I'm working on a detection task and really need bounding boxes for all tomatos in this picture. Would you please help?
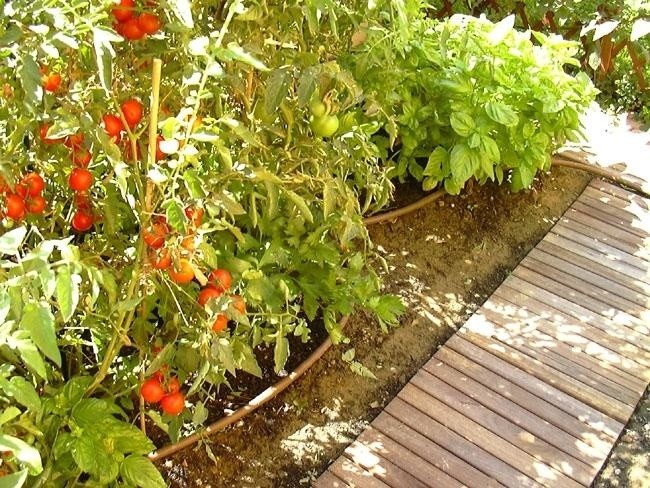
[0,0,248,415]
[307,97,339,140]
